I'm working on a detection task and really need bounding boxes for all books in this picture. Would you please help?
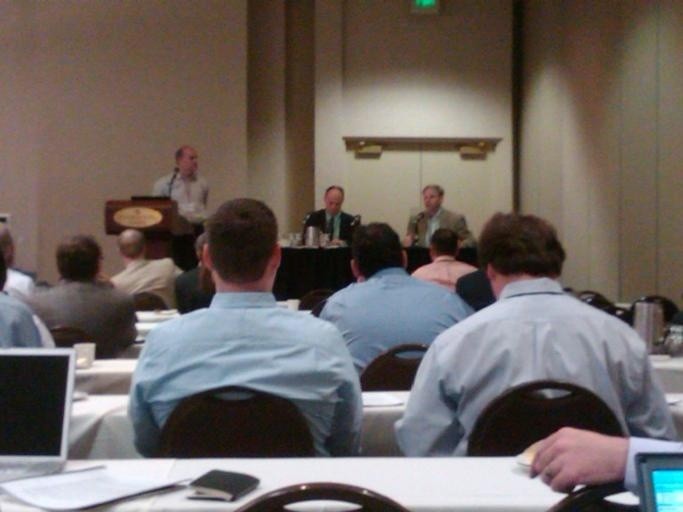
[188,469,259,502]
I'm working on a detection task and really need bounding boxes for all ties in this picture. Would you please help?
[633,451,683,511]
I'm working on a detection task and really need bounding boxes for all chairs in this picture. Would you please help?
[0,239,683,512]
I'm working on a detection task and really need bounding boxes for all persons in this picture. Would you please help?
[404,184,473,247]
[149,147,210,234]
[302,186,354,247]
[173,235,217,314]
[412,228,476,293]
[0,222,42,349]
[529,427,683,496]
[28,232,138,361]
[319,222,476,377]
[111,230,180,310]
[394,209,676,457]
[128,198,363,459]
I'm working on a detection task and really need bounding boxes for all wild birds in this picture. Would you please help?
[0,348,77,483]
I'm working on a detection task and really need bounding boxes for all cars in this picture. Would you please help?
[305,225,319,248]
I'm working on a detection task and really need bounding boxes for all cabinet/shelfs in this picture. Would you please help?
[633,295,665,354]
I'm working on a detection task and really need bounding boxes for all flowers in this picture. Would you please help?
[300,208,311,250]
[350,214,363,246]
[168,166,180,200]
[412,211,425,246]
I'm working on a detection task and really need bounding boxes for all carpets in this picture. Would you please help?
[326,216,334,241]
